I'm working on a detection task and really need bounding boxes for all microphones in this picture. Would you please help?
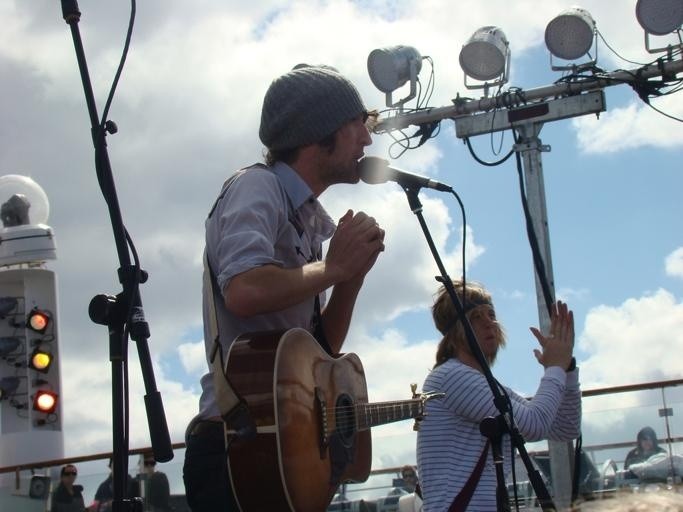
[357,154,453,194]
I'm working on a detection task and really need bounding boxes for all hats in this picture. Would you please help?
[259,63,368,151]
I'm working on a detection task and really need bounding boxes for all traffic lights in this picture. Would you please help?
[27,309,58,431]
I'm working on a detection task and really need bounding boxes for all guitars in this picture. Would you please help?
[222,327,445,511]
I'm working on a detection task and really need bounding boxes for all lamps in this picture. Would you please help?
[366,1,683,109]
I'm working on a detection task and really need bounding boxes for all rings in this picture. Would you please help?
[548,334,554,338]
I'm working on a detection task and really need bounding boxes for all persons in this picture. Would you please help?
[181,64,387,511]
[93,457,136,498]
[50,464,84,512]
[400,463,417,483]
[625,425,668,479]
[133,452,168,502]
[414,278,581,512]
